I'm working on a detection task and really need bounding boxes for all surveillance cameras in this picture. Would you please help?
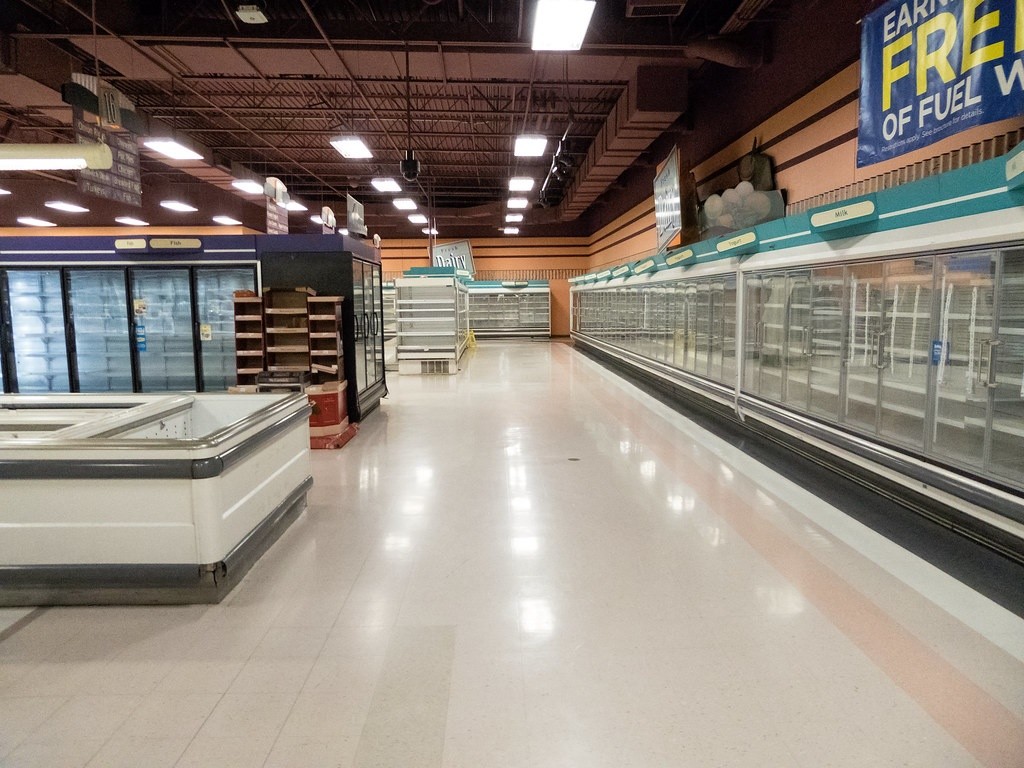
[400,160,420,181]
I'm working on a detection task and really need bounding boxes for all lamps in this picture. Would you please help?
[1,1,596,238]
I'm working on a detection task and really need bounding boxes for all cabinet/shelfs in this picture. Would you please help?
[230,287,349,435]
